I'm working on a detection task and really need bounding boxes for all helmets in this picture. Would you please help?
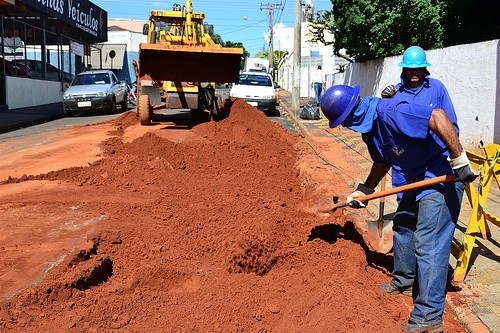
[321,82,360,128]
[398,46,431,68]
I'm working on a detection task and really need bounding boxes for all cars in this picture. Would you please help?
[11,59,74,83]
[229,71,281,115]
[0,57,31,78]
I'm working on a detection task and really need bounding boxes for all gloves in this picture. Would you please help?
[447,150,478,184]
[381,84,397,98]
[346,183,375,209]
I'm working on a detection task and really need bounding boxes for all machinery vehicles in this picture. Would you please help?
[132,0,243,124]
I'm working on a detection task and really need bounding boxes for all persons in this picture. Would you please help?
[382,46,460,206]
[311,65,326,106]
[321,85,478,333]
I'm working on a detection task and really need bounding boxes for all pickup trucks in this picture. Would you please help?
[62,69,128,116]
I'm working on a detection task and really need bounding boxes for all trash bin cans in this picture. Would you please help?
[314,82,322,104]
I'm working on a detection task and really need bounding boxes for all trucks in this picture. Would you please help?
[245,57,270,85]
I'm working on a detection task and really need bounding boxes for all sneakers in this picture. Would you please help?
[377,283,413,296]
[401,320,443,333]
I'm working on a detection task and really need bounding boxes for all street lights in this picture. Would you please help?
[244,16,270,28]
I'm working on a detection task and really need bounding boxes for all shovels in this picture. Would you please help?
[318,173,457,213]
[367,173,394,255]
